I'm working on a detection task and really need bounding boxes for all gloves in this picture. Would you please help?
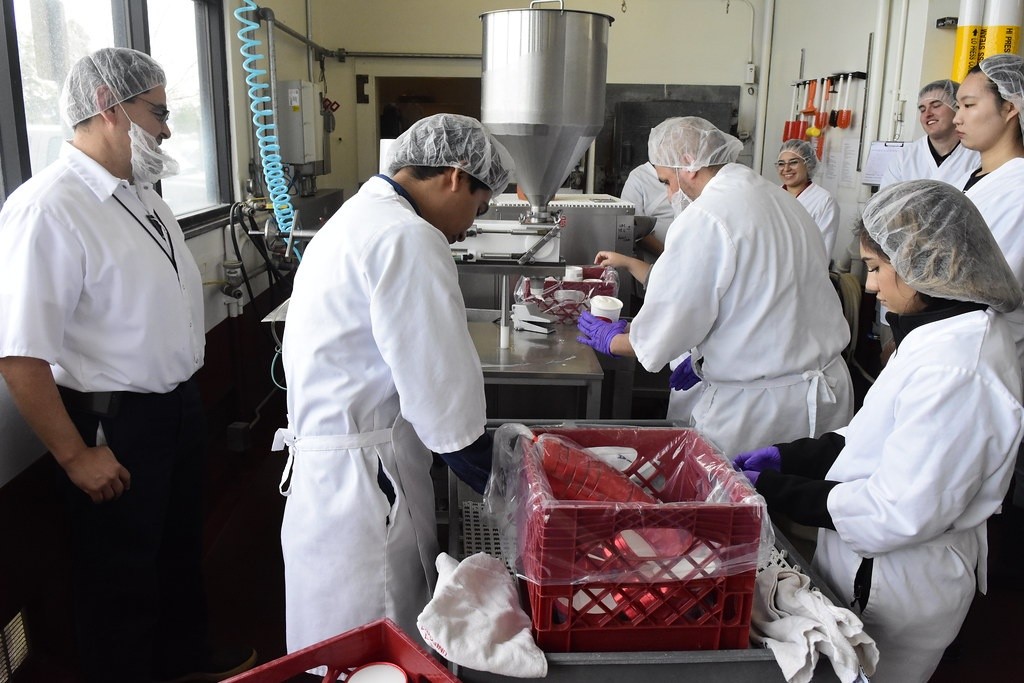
[731,446,782,487]
[576,312,627,360]
[669,355,701,390]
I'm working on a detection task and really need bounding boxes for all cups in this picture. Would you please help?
[344,662,408,683]
[589,295,624,323]
[564,265,583,282]
[583,278,603,282]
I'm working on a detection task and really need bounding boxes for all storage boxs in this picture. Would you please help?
[218,618,464,683]
[525,266,615,323]
[522,428,763,654]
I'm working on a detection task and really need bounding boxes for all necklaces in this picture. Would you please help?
[109,187,179,275]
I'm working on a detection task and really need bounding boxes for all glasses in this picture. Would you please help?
[133,96,169,124]
[774,158,802,170]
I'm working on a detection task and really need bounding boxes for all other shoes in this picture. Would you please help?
[171,641,257,683]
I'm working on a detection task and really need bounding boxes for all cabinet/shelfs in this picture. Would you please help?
[449,321,605,556]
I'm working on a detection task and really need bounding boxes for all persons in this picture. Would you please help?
[279,112,510,683]
[876,77,982,372]
[771,138,840,271]
[951,54,1024,300]
[620,150,696,270]
[730,179,1024,683]
[1,47,260,683]
[578,116,853,483]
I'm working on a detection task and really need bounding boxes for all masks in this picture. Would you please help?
[669,166,693,220]
[118,103,179,186]
